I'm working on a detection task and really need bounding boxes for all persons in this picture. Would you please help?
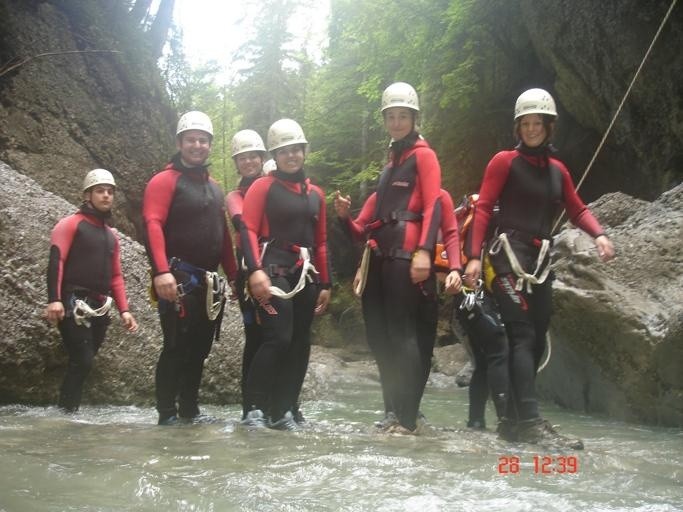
[464,88,617,448]
[334,132,464,425]
[45,169,139,413]
[140,109,239,423]
[238,119,331,429]
[226,130,306,420]
[354,81,440,434]
[452,194,512,431]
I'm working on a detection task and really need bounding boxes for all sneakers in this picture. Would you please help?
[240,403,310,434]
[373,410,437,437]
[463,414,584,452]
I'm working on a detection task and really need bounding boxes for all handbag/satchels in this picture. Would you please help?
[434,194,479,270]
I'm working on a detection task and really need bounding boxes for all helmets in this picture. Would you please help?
[381,83,420,111]
[267,119,309,153]
[514,88,557,121]
[176,111,213,137]
[82,169,116,192]
[231,130,266,157]
